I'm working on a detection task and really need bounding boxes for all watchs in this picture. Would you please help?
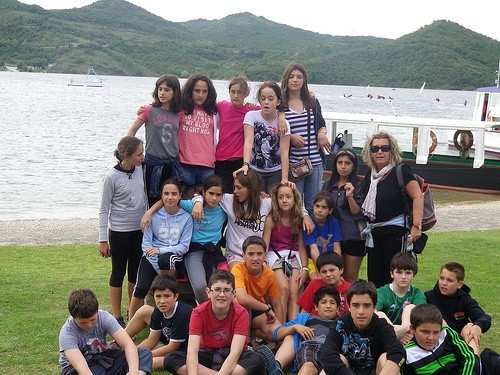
[413,224,423,230]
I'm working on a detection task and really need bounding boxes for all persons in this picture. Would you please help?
[211,78,289,189]
[163,270,265,375]
[319,278,407,375]
[58,289,154,375]
[232,80,296,194]
[402,303,484,375]
[358,130,424,287]
[374,251,427,327]
[127,74,182,206]
[230,234,282,342]
[132,273,195,372]
[271,286,343,375]
[139,168,343,308]
[423,261,493,357]
[275,62,332,223]
[296,251,353,322]
[137,73,221,199]
[322,147,369,284]
[96,135,150,331]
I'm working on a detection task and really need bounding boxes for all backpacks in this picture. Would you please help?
[397,163,436,231]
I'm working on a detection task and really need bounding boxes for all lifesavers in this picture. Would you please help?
[454,130,473,152]
[413,130,438,154]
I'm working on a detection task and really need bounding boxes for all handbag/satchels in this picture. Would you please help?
[288,157,314,180]
[282,259,293,278]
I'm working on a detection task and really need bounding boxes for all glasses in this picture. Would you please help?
[210,287,233,294]
[370,145,392,153]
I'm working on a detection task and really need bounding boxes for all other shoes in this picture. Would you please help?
[116,317,126,327]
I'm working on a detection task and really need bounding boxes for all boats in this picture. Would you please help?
[67,66,102,87]
[323,62,500,196]
